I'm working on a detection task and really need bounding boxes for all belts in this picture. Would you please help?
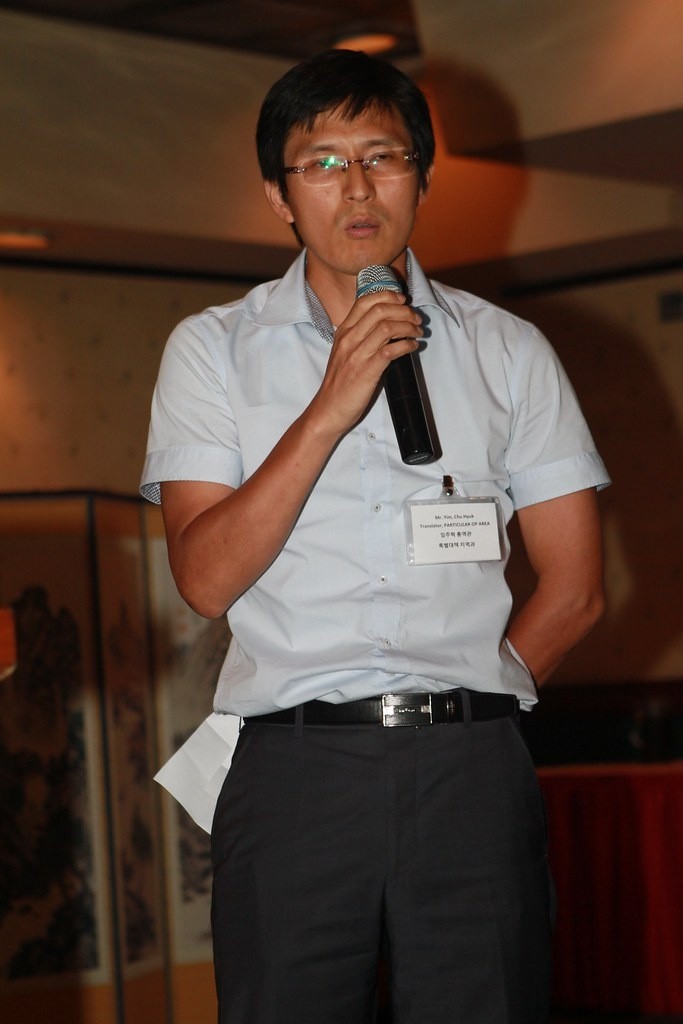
[242,687,521,731]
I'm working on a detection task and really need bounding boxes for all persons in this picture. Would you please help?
[140,50,612,1024]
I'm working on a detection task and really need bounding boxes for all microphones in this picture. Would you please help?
[356,265,434,464]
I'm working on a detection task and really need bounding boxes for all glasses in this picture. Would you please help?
[275,150,422,187]
